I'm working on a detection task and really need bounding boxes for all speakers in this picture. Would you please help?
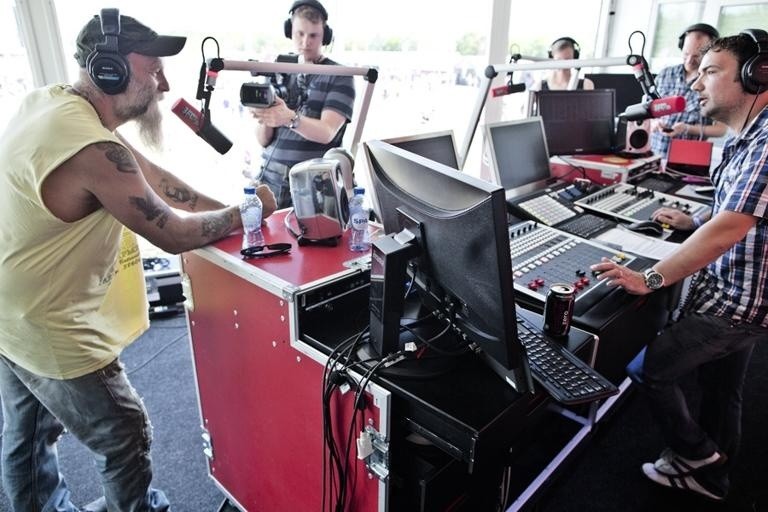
[614,115,652,155]
[286,156,352,245]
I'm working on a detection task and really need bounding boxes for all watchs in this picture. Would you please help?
[643,268,666,291]
[286,115,300,129]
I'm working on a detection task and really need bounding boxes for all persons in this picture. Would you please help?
[0,14,279,512]
[529,38,595,91]
[641,23,729,159]
[249,0,356,209]
[590,34,768,501]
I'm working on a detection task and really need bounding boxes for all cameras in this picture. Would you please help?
[239,53,312,110]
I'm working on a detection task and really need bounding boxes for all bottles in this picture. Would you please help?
[348,187,370,252]
[239,187,265,254]
[542,283,576,338]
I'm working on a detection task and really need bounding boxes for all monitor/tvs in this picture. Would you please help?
[355,140,536,396]
[379,129,461,172]
[537,88,617,155]
[483,116,554,201]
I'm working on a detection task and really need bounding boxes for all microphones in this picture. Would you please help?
[545,175,558,184]
[171,96,233,155]
[491,82,527,99]
[621,96,686,126]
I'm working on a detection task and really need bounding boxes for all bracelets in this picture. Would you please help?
[693,215,701,228]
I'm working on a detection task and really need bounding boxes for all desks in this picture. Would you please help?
[180,154,662,511]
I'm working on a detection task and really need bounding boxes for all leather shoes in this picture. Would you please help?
[652,448,728,478]
[641,461,729,503]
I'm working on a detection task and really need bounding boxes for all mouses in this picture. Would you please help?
[627,220,664,238]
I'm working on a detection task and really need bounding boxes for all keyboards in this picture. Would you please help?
[516,311,621,405]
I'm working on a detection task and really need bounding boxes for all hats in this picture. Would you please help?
[72,14,189,68]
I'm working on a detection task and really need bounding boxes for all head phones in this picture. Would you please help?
[677,23,719,49]
[85,8,131,95]
[739,28,768,95]
[282,0,333,47]
[547,37,580,59]
